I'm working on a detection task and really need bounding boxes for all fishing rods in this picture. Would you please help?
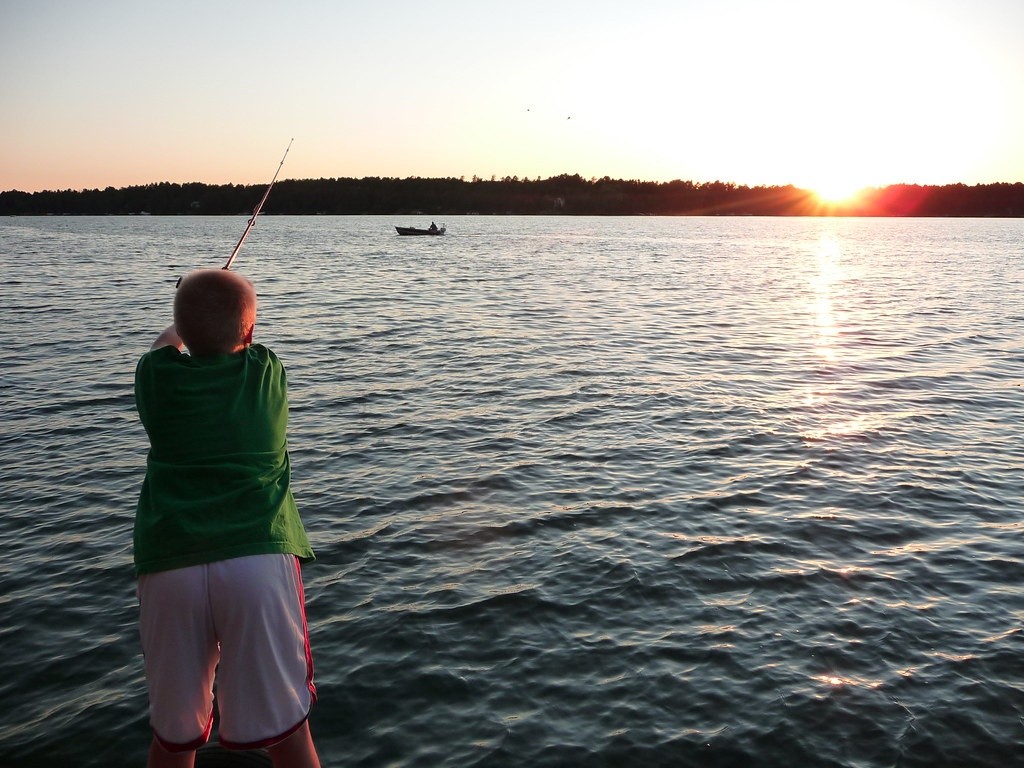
[222,137,294,274]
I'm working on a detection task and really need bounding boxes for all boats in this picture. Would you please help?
[394,226,446,236]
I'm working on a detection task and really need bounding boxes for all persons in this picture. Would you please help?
[132,268,321,768]
[429,222,438,231]
